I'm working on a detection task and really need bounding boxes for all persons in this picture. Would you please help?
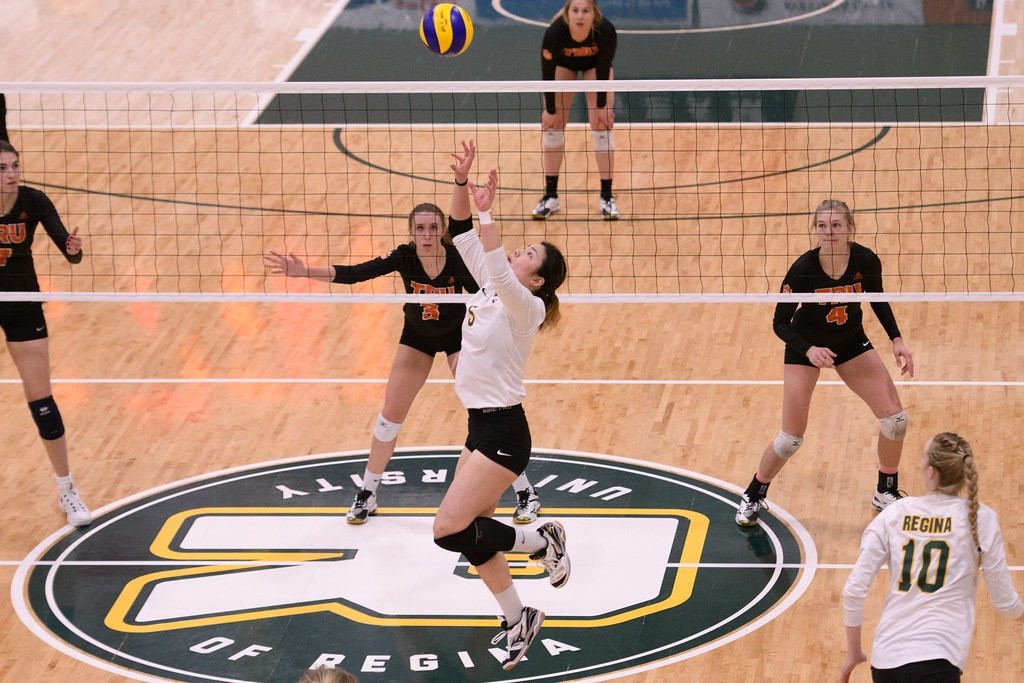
[531,0,621,220]
[735,199,913,526]
[0,93,92,527]
[837,432,1024,683]
[263,203,541,524]
[433,139,572,671]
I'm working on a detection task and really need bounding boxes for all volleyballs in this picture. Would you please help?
[419,3,475,57]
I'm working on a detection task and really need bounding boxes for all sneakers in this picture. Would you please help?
[513,486,541,524]
[346,486,377,524]
[532,196,560,219]
[871,483,909,511]
[600,196,620,219]
[735,492,769,527]
[529,520,571,589]
[491,606,545,671]
[59,490,92,526]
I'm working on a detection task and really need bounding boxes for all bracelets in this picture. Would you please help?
[475,208,496,225]
[455,178,469,187]
